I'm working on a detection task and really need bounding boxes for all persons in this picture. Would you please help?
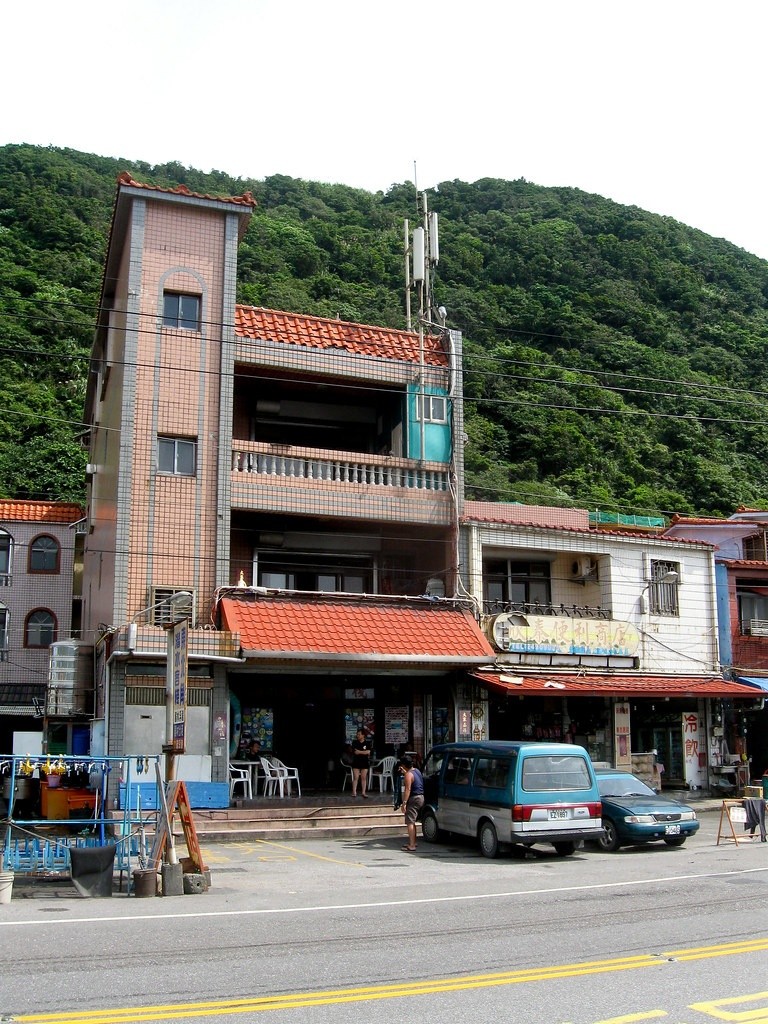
[399,755,425,852]
[351,729,371,798]
[244,739,274,795]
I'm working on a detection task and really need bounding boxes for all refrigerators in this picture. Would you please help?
[653,727,689,786]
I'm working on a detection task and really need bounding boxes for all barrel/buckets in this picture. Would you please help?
[45,637,97,717]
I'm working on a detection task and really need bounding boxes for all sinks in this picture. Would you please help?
[711,764,739,775]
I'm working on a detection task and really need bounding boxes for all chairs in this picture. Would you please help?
[340,759,369,793]
[271,757,302,799]
[260,757,289,799]
[367,757,397,794]
[229,764,252,800]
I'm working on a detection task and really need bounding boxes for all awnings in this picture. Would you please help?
[737,676,768,691]
[467,673,768,700]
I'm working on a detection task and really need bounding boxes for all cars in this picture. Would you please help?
[545,768,701,852]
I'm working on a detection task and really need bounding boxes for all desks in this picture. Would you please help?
[230,760,262,798]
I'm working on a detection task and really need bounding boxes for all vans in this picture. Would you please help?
[399,738,605,858]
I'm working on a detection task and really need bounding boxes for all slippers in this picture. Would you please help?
[401,844,418,851]
[351,792,369,798]
